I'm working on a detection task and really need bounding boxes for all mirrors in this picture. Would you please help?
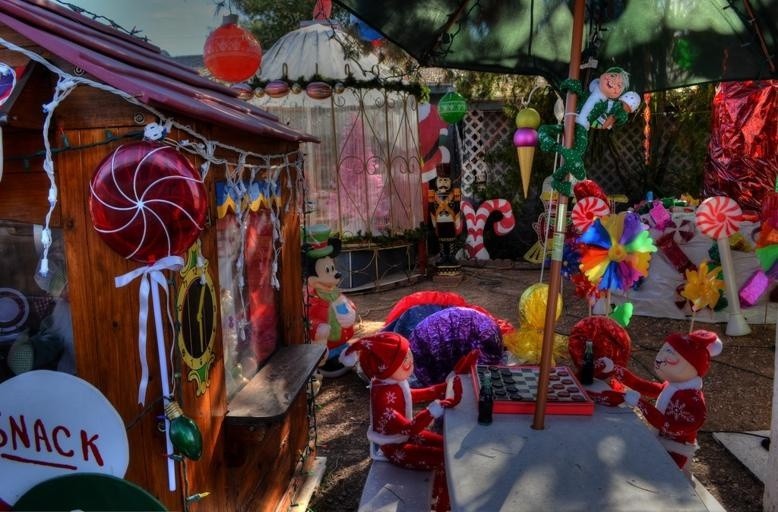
[177,239,219,398]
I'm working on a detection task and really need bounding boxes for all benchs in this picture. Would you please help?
[684,469,727,512]
[356,460,438,512]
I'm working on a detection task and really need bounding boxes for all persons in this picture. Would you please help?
[537,65,629,195]
[429,164,464,275]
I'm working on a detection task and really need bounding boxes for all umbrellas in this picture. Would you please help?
[333,0,778,430]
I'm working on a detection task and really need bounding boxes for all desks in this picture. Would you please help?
[444,362,710,512]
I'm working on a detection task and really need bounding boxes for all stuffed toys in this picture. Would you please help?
[595,330,726,471]
[342,330,464,511]
[302,223,360,378]
